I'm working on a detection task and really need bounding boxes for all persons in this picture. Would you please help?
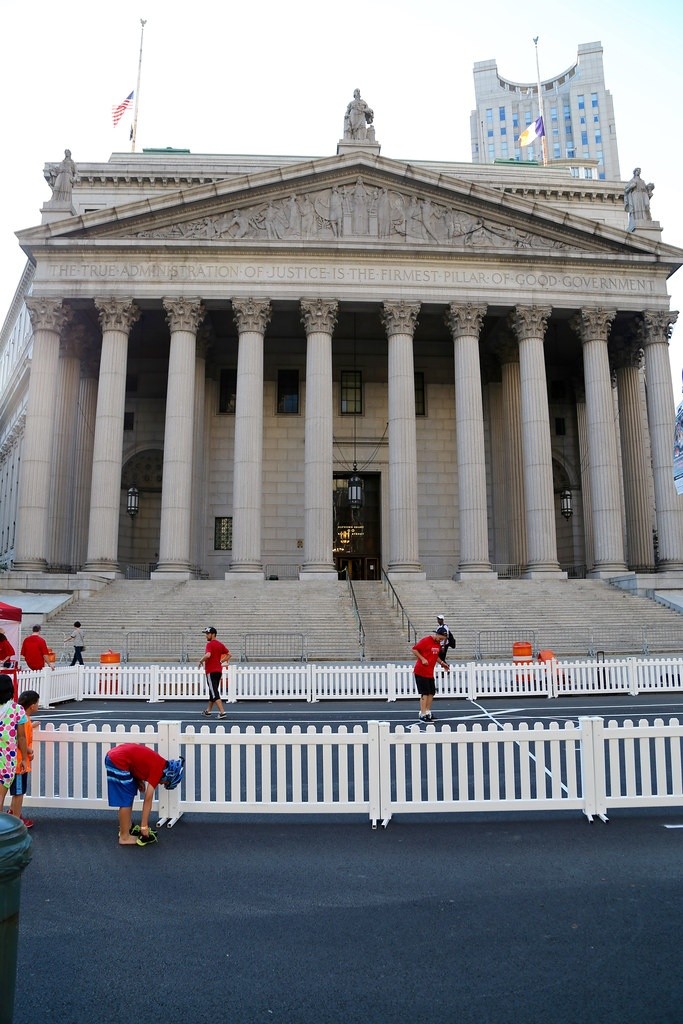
[197,627,232,719]
[344,88,374,140]
[105,742,185,845]
[0,633,15,669]
[412,628,451,722]
[64,621,86,665]
[436,615,449,668]
[7,690,40,827]
[624,167,655,224]
[0,674,27,812]
[50,148,77,204]
[20,624,55,671]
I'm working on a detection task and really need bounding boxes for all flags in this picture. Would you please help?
[112,90,135,128]
[518,116,545,147]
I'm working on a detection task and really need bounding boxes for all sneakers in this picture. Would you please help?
[136,831,159,846]
[129,825,157,836]
[214,712,227,719]
[201,710,211,717]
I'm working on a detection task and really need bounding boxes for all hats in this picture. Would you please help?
[437,615,445,619]
[202,627,217,634]
[0,628,5,635]
[436,627,447,637]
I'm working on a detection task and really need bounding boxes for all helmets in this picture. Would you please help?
[160,756,185,790]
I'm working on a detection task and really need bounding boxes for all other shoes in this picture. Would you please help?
[5,809,34,828]
[427,714,439,722]
[419,715,434,724]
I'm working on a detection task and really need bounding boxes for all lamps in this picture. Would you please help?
[560,376,574,522]
[348,311,363,510]
[127,395,140,520]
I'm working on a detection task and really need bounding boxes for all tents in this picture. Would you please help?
[0,602,22,670]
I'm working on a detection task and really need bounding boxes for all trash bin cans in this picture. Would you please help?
[511,641,534,685]
[99,649,122,695]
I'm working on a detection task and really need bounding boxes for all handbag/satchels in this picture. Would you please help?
[80,646,85,651]
[442,624,456,649]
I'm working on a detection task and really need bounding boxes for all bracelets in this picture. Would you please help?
[441,661,443,664]
[141,826,147,830]
